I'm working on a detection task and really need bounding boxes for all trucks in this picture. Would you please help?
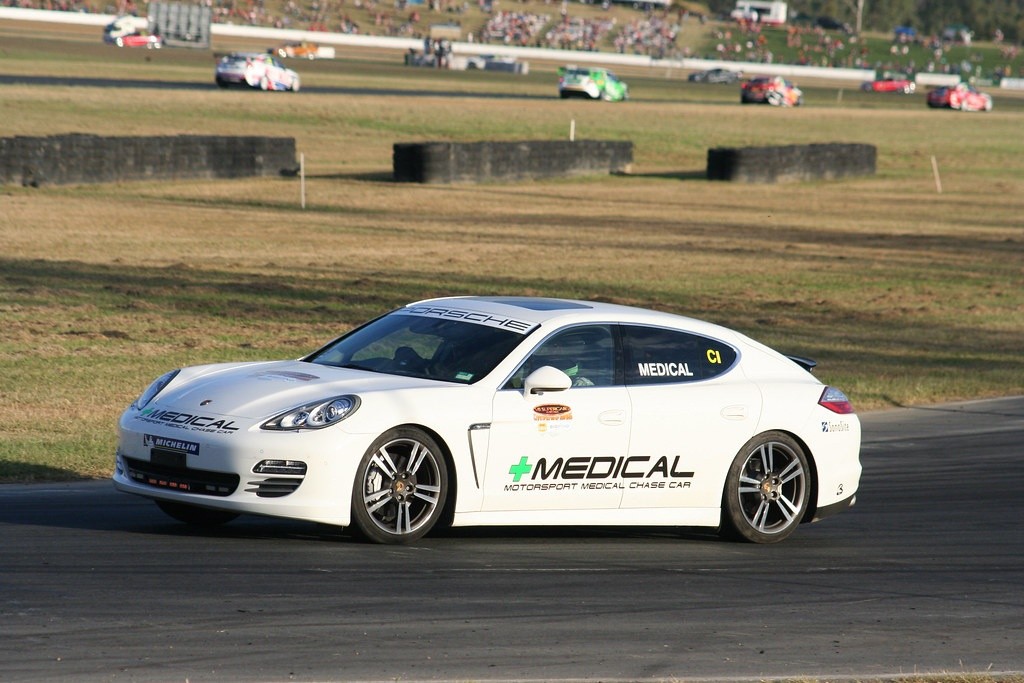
[729,0,787,26]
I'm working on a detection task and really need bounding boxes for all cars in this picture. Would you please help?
[405,49,485,71]
[113,295,865,542]
[273,44,318,60]
[214,50,302,93]
[687,68,737,86]
[861,75,914,95]
[105,29,162,49]
[742,75,804,105]
[926,82,993,112]
[558,66,630,102]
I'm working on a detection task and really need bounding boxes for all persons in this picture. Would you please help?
[504,334,595,387]
[0,1,1024,78]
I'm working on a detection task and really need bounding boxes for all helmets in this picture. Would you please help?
[529,333,579,380]
